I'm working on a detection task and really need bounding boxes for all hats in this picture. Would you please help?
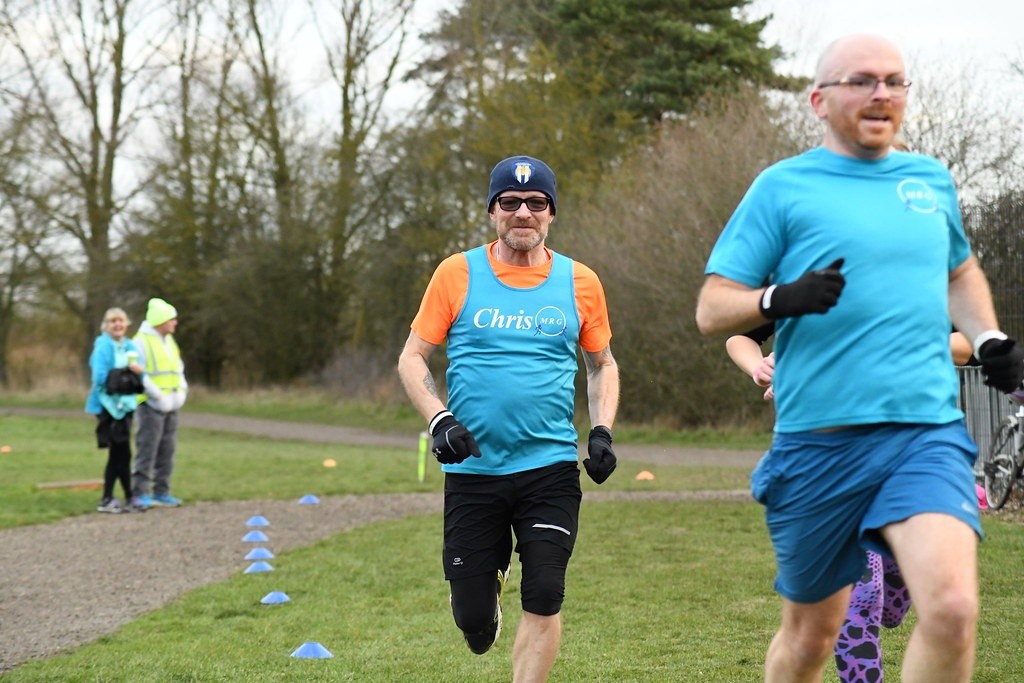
[487,155,556,215]
[146,297,177,327]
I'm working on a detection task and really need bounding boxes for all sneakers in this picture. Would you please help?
[97,497,130,513]
[125,496,148,513]
[141,495,163,507]
[153,494,184,507]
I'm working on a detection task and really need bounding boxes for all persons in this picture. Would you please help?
[88,305,150,515]
[127,298,191,513]
[722,276,972,683]
[396,150,621,682]
[693,32,1021,682]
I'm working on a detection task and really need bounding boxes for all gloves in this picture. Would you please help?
[973,330,1024,394]
[759,257,846,317]
[582,425,616,485]
[427,410,482,464]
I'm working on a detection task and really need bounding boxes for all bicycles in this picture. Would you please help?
[983,389,1024,510]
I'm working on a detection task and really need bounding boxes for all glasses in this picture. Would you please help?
[817,73,913,95]
[496,196,551,211]
